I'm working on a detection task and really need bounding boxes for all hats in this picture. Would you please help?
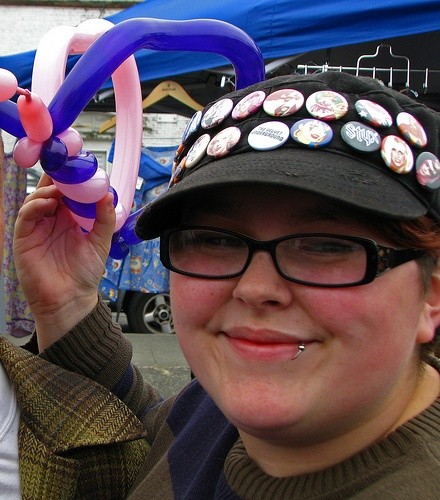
[135,70,439,241]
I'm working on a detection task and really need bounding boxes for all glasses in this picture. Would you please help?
[157,223,430,288]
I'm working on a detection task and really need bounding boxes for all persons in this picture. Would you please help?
[0,71,440,500]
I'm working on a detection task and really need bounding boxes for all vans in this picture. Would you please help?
[1,101,186,333]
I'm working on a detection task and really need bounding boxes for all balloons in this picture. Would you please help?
[0,17,267,263]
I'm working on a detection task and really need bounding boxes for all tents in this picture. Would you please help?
[1,0,439,122]
